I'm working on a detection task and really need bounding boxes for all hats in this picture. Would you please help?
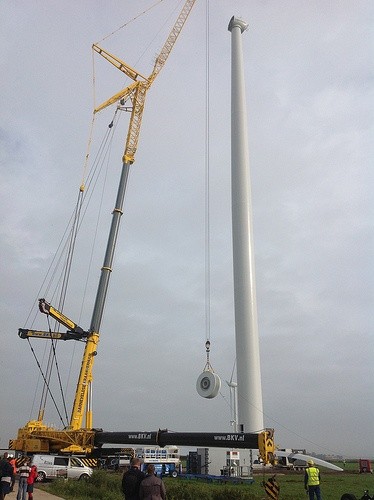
[307,460,314,464]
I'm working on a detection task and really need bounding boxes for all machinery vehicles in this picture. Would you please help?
[1,1,205,458]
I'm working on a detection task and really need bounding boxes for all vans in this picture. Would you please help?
[28,453,94,482]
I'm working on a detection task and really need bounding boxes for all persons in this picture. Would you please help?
[139,464,167,500]
[304,459,320,500]
[122,459,144,500]
[0,452,38,500]
[341,494,357,500]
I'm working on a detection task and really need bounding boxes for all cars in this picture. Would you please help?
[251,459,273,472]
